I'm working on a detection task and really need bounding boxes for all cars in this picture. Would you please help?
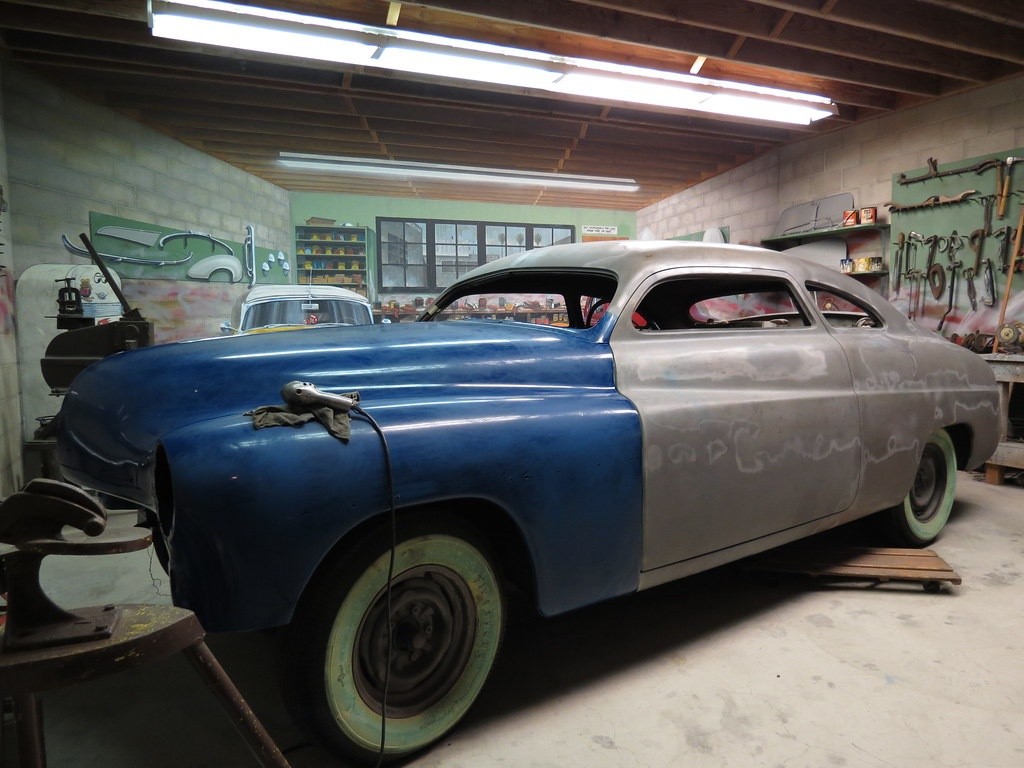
[44,239,1003,764]
[220,284,391,335]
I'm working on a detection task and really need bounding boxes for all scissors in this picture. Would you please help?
[981,195,996,236]
[962,267,976,311]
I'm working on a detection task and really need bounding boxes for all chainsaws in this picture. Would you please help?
[995,321,1024,346]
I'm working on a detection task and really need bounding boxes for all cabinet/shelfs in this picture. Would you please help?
[761,222,889,278]
[975,353,1024,485]
[294,221,369,306]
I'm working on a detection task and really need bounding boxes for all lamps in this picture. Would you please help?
[145,0,838,193]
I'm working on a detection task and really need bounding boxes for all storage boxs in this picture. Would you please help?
[304,217,337,227]
